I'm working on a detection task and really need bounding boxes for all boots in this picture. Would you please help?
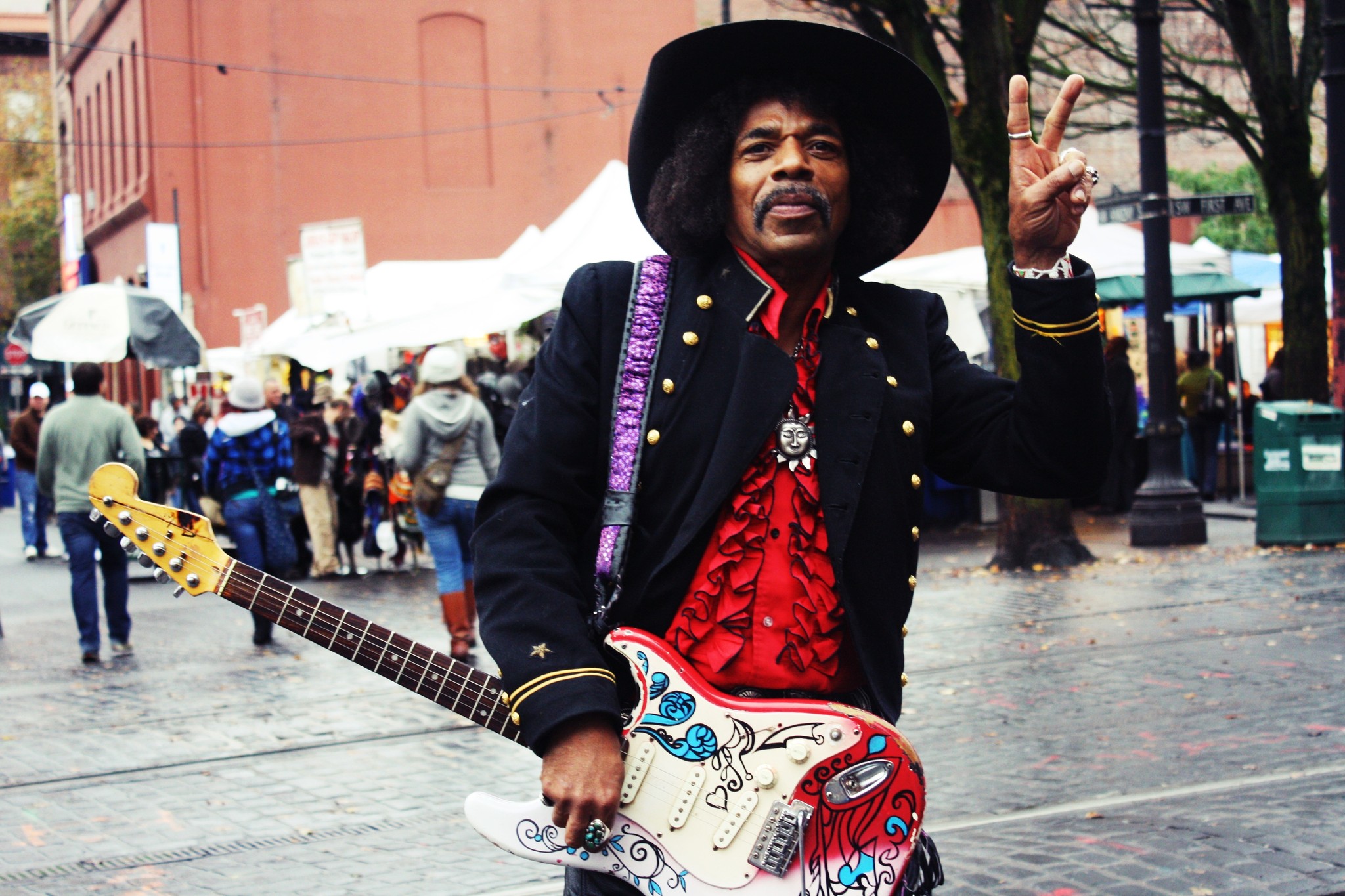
[464,578,478,647]
[438,591,470,662]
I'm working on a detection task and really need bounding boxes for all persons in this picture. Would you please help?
[471,18,1093,896]
[1086,336,1139,526]
[1232,345,1288,442]
[11,381,52,561]
[201,370,299,646]
[33,364,144,665]
[1175,350,1226,504]
[392,347,501,657]
[137,329,524,576]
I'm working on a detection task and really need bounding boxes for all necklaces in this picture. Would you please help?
[764,338,817,474]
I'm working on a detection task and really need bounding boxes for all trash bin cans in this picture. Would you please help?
[1253,401,1345,548]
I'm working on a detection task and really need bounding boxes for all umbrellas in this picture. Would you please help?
[6,278,202,369]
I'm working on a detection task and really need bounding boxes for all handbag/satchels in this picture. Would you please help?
[252,470,298,574]
[413,459,454,517]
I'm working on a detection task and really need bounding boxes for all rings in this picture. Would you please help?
[1008,130,1033,141]
[1059,147,1077,166]
[539,791,552,807]
[1086,166,1100,186]
[584,819,611,849]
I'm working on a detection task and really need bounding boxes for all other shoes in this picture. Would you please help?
[24,545,37,560]
[81,650,99,662]
[252,641,289,656]
[38,548,61,558]
[110,639,132,657]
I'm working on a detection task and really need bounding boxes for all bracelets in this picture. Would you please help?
[1013,253,1077,280]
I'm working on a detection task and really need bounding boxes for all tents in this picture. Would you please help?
[492,156,669,309]
[859,193,1334,360]
[247,258,558,373]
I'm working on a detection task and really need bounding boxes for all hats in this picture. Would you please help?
[29,381,51,399]
[493,375,523,409]
[420,346,466,384]
[627,14,951,277]
[226,374,266,410]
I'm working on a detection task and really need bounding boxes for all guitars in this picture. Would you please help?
[78,462,926,896]
